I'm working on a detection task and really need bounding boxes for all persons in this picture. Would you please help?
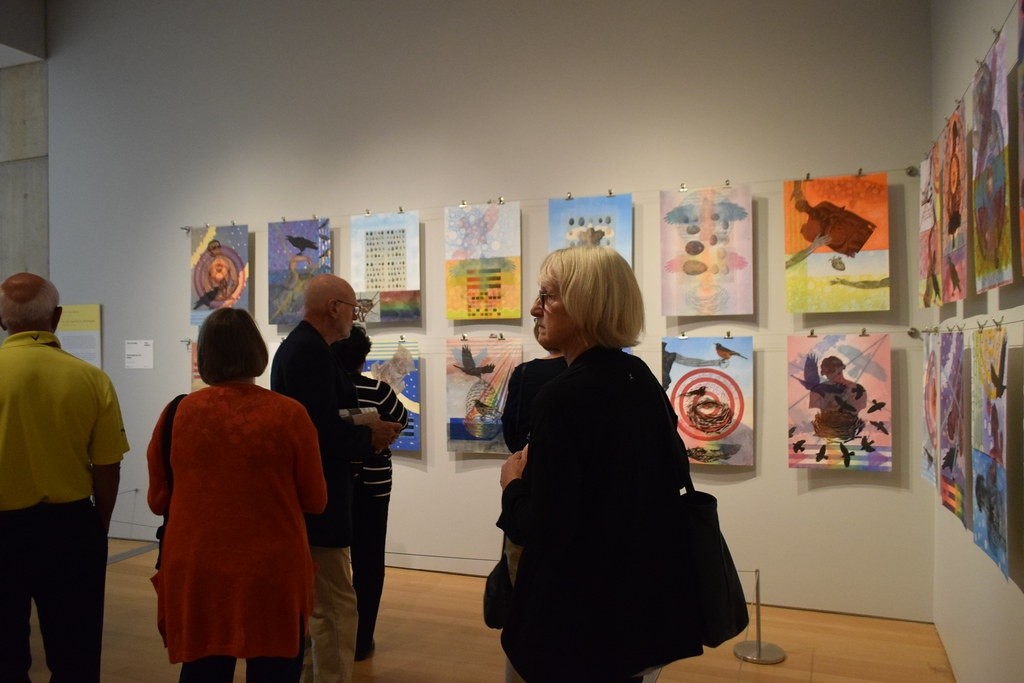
[146,247,691,683]
[0,273,132,683]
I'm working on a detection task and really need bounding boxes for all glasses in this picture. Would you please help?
[538,290,559,308]
[328,298,361,316]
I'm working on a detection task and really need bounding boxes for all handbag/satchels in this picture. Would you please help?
[156,394,187,570]
[631,356,749,647]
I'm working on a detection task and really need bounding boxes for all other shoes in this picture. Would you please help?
[356,637,375,661]
